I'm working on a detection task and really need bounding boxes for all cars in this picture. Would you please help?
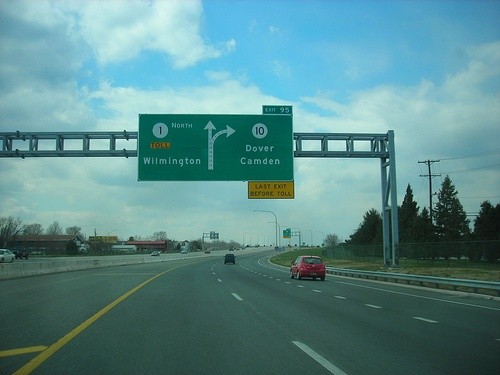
[290,255,326,281]
[180,250,188,254]
[223,253,237,265]
[0,248,16,264]
[150,250,161,256]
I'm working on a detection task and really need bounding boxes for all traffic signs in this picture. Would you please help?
[136,112,295,183]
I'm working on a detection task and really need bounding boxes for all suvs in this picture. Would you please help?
[12,245,30,260]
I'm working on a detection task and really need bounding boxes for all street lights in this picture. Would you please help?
[268,221,281,248]
[107,229,119,235]
[252,209,277,250]
